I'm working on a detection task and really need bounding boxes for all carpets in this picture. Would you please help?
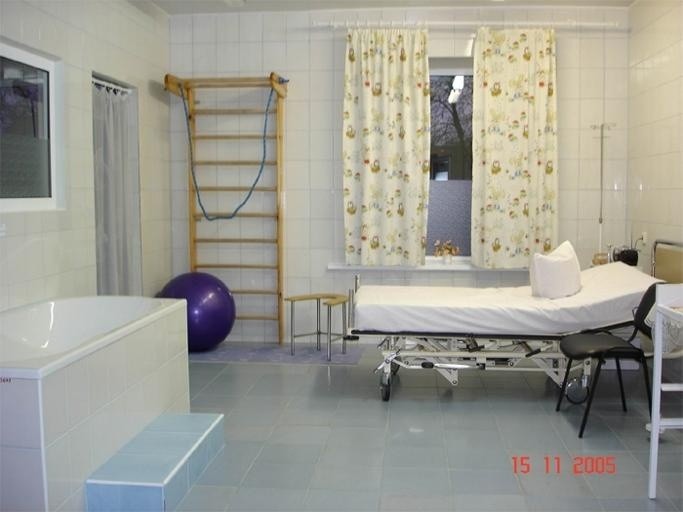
[190,342,363,365]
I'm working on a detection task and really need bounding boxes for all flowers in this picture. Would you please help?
[432,240,458,256]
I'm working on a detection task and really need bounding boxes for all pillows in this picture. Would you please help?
[530,241,580,297]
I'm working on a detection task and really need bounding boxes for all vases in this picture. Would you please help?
[443,255,453,265]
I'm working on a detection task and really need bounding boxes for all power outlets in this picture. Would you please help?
[641,232,646,244]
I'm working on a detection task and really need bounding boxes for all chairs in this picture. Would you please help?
[557,281,668,440]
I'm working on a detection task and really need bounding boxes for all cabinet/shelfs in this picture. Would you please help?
[600,267,643,371]
[645,283,683,499]
[0,303,227,512]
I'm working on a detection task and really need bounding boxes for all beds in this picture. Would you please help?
[342,240,682,402]
